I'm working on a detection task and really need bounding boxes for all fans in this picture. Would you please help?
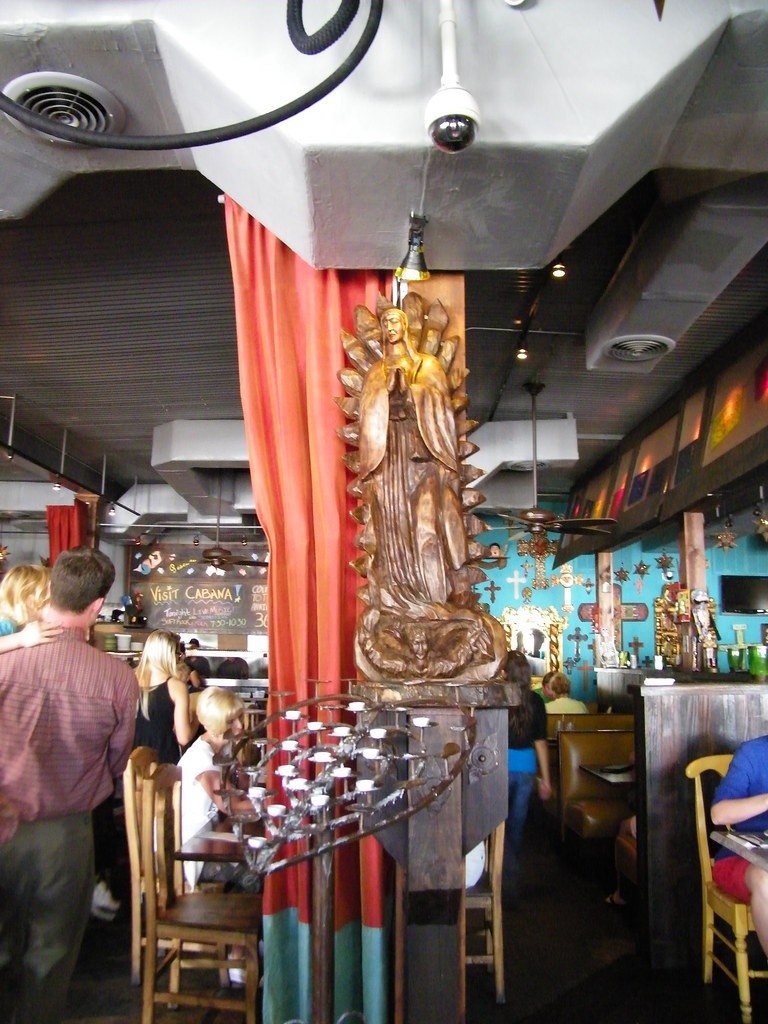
[478,383,617,537]
[168,472,268,569]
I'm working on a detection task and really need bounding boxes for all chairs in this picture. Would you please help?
[464,821,506,1001]
[124,746,232,1009]
[685,754,767,1023]
[139,762,261,1024]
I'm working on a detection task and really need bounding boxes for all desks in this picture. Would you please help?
[710,831,768,872]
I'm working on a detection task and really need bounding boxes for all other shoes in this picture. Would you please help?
[226,965,262,989]
[90,881,121,911]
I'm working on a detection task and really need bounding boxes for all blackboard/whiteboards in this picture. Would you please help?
[122,545,270,636]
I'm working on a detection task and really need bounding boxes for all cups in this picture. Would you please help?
[728,649,746,672]
[104,634,144,652]
[748,646,768,677]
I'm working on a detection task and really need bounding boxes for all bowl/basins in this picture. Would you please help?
[413,716,430,727]
[363,748,379,758]
[333,767,351,776]
[289,778,309,787]
[370,728,387,738]
[278,765,296,773]
[308,721,323,729]
[282,740,298,749]
[267,804,287,816]
[314,751,331,759]
[349,702,365,710]
[334,727,350,735]
[356,780,375,790]
[286,710,301,718]
[248,837,267,849]
[312,794,331,806]
[248,786,266,798]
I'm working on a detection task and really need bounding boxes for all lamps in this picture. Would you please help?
[396,217,429,284]
[109,501,118,516]
[52,482,62,491]
[193,534,200,547]
[425,0,481,156]
[241,535,247,546]
[135,537,141,545]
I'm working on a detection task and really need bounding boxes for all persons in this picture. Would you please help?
[184,639,211,678]
[357,308,468,617]
[152,687,267,989]
[0,546,139,1024]
[132,629,199,766]
[358,623,489,678]
[217,657,249,680]
[710,735,768,960]
[533,672,590,714]
[604,751,637,906]
[0,565,65,654]
[494,650,551,910]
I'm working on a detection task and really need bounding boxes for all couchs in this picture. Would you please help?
[614,835,638,882]
[557,731,636,839]
[533,713,635,816]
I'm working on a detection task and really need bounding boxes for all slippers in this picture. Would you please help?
[603,895,631,908]
